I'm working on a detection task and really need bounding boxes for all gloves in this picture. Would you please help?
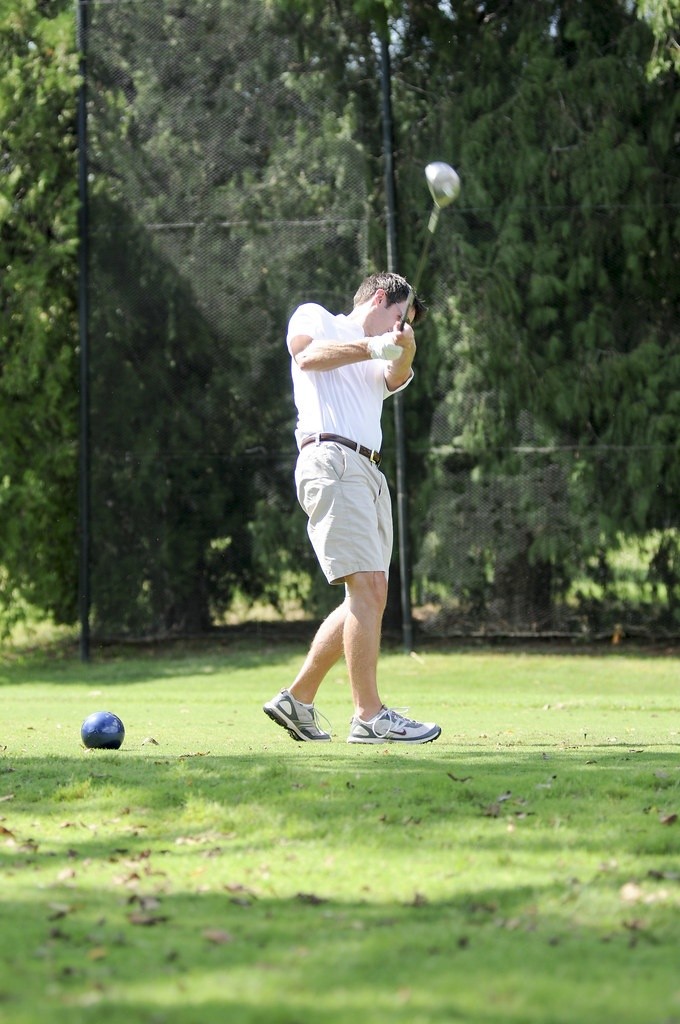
[368,331,405,362]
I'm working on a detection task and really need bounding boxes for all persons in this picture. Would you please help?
[263,271,442,743]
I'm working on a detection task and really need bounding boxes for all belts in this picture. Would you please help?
[300,433,383,467]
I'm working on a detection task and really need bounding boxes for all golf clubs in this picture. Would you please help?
[398,163,461,334]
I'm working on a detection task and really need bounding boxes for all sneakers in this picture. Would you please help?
[347,704,442,744]
[263,688,333,743]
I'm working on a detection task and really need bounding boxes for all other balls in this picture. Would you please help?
[81,711,125,751]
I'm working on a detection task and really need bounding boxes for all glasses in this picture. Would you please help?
[395,302,411,325]
